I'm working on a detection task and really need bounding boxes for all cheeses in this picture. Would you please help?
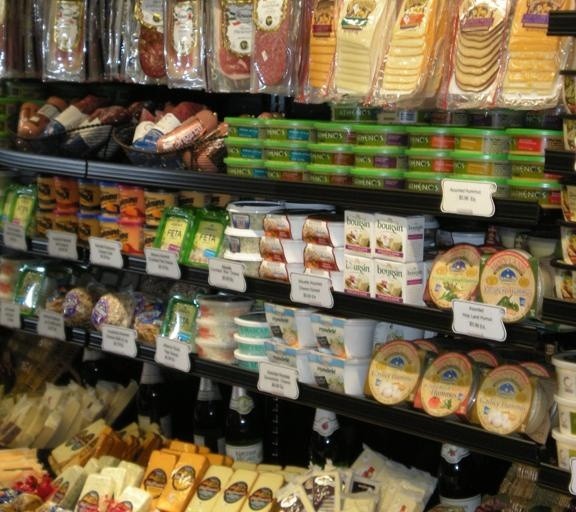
[0,379,429,512]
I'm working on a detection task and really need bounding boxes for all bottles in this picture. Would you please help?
[307,407,350,469]
[193,376,263,465]
[438,443,484,510]
[137,361,174,439]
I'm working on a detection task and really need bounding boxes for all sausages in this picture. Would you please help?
[16,94,286,174]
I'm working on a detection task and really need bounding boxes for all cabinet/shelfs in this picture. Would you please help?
[1,0,575,512]
